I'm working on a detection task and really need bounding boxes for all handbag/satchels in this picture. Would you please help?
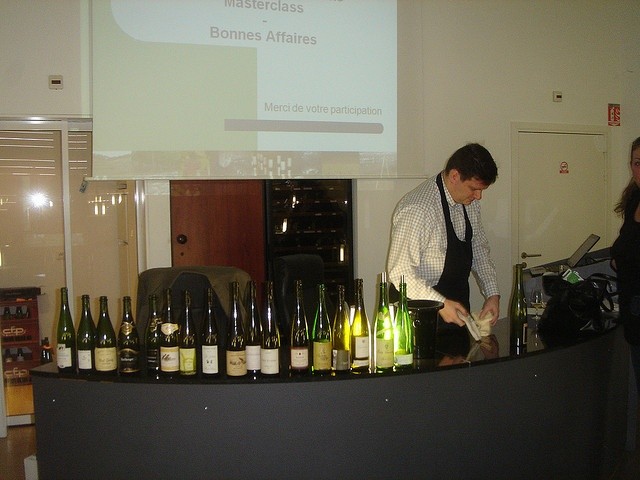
[538,272,620,348]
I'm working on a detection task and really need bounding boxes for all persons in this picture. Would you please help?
[387,142,502,355]
[611,136,639,386]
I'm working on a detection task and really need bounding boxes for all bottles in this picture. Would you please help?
[179,288,200,378]
[260,281,280,377]
[310,283,333,375]
[349,279,371,375]
[93,296,118,375]
[392,274,414,371]
[56,287,77,375]
[3,306,12,343]
[14,307,25,341]
[3,349,14,384]
[16,348,26,383]
[198,288,224,378]
[243,281,261,377]
[331,285,351,378]
[370,272,394,375]
[118,296,142,376]
[510,346,527,357]
[225,282,248,378]
[509,264,528,347]
[158,287,182,377]
[289,280,313,376]
[76,295,96,376]
[144,293,164,377]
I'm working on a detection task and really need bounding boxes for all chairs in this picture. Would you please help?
[269,261,303,340]
[132,265,262,360]
[273,254,335,326]
